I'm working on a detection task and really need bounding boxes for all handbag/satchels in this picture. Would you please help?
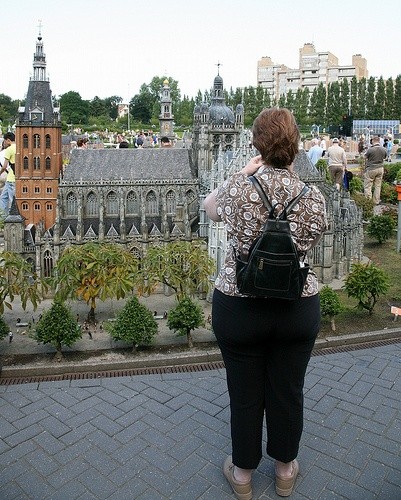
[8,161,15,176]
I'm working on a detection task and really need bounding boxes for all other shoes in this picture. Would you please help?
[274,459,299,496]
[223,455,254,500]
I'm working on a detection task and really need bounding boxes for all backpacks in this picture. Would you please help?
[233,175,312,301]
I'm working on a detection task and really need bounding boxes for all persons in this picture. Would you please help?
[205,108,328,500]
[0,128,395,215]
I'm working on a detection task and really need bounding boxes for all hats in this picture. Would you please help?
[333,138,340,143]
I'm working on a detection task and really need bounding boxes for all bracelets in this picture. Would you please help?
[344,166,347,169]
